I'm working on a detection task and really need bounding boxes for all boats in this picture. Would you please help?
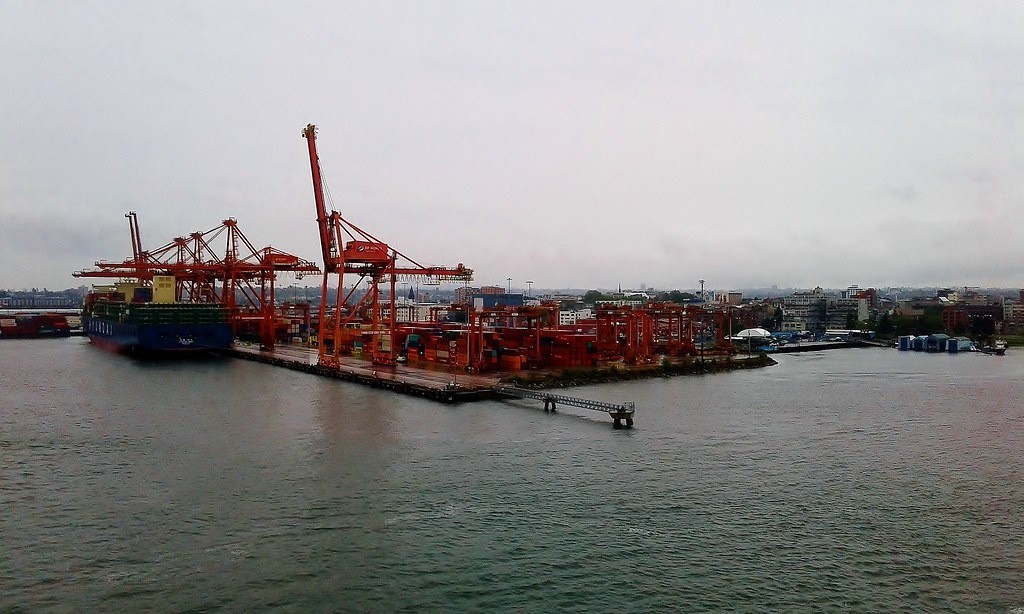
[0,312,70,338]
[79,276,233,355]
[996,340,1008,348]
[982,345,1006,355]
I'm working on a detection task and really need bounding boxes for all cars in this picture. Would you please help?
[395,357,406,362]
[808,337,816,342]
[832,339,842,342]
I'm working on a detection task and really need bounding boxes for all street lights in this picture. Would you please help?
[401,283,408,321]
[896,292,898,302]
[305,285,308,299]
[463,302,470,366]
[526,282,533,296]
[700,280,705,364]
[506,277,512,294]
[293,283,299,337]
